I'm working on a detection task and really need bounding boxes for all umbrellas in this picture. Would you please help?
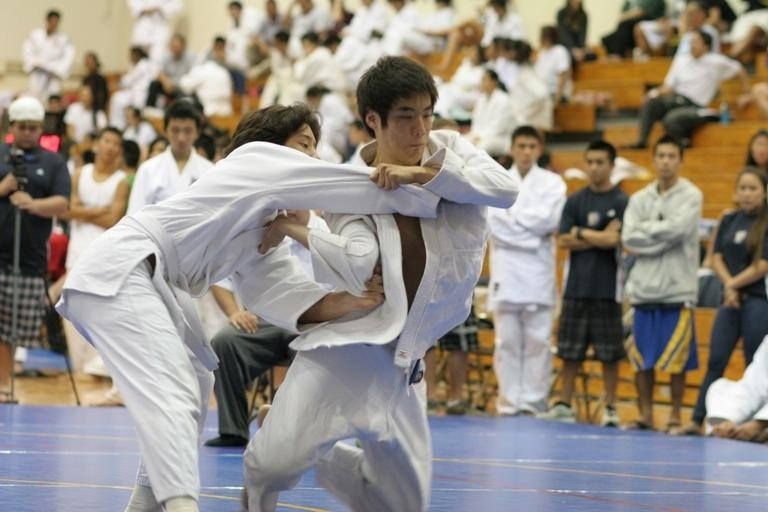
[206,432,246,450]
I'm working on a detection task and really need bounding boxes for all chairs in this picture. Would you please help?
[601,405,619,429]
[535,403,576,425]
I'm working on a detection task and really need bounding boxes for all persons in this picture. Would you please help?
[145,35,193,109]
[255,1,291,51]
[218,2,254,62]
[202,37,227,67]
[666,168,767,436]
[282,0,327,58]
[673,1,722,60]
[723,0,768,72]
[704,334,767,443]
[321,35,376,87]
[348,121,370,158]
[367,0,421,58]
[0,96,75,404]
[621,137,702,437]
[297,30,348,93]
[65,83,107,142]
[403,0,456,59]
[179,52,235,118]
[557,0,587,66]
[54,100,446,508]
[485,124,568,419]
[128,0,183,55]
[64,128,130,273]
[78,52,109,110]
[449,69,517,158]
[20,9,73,97]
[474,0,526,65]
[257,30,301,111]
[110,49,158,133]
[128,102,216,216]
[305,84,355,164]
[601,0,669,60]
[532,25,574,113]
[556,139,629,427]
[239,56,520,507]
[620,30,752,150]
[742,130,768,175]
[342,2,391,44]
[504,40,554,136]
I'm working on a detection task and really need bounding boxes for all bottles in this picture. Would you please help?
[8,97,43,122]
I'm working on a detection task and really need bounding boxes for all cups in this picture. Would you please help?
[18,124,37,132]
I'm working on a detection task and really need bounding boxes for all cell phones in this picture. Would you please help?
[4,148,39,180]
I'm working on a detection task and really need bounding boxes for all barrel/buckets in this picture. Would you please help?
[0,391,17,404]
[664,421,680,436]
[625,419,653,430]
